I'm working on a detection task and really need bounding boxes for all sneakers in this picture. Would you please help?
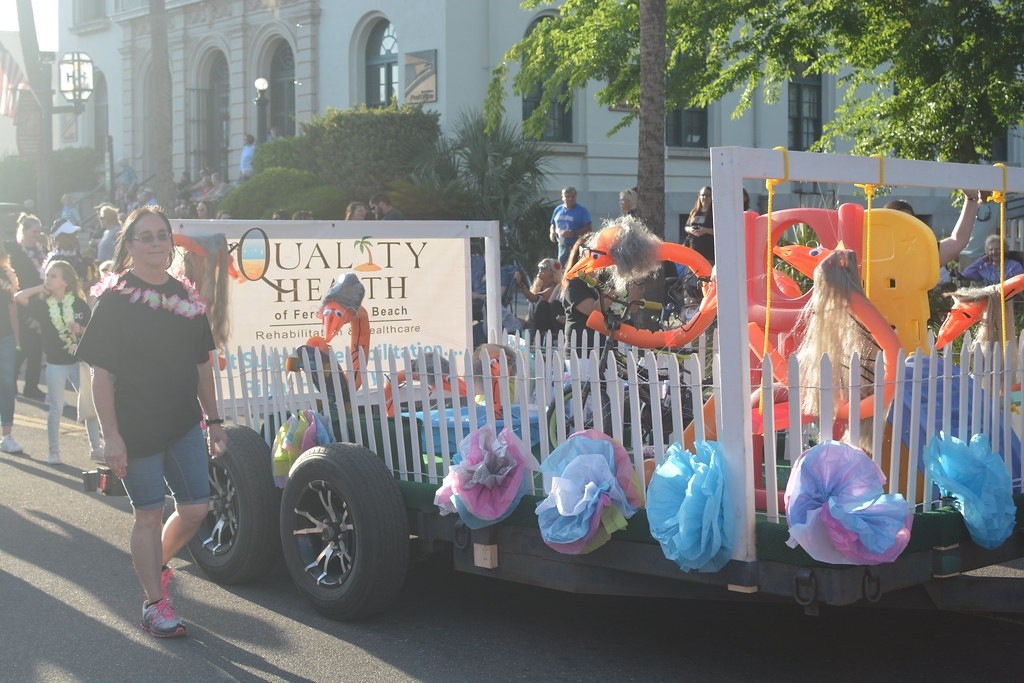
[140,599,186,637]
[160,564,173,605]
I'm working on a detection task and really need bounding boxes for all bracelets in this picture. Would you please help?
[966,197,976,201]
[203,418,224,425]
[519,282,526,289]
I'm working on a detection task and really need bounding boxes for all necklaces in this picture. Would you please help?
[85,266,208,314]
[0,263,20,293]
[45,291,80,353]
[23,241,47,279]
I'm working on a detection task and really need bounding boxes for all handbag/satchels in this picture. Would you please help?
[76,361,96,421]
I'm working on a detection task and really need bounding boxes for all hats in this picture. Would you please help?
[51,220,81,238]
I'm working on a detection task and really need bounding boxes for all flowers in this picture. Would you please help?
[271,409,1021,566]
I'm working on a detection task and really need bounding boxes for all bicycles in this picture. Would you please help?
[542,270,714,450]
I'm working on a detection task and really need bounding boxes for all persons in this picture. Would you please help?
[116,126,284,207]
[472,252,564,349]
[619,189,639,217]
[960,234,1024,286]
[273,209,291,221]
[683,186,716,299]
[883,187,979,267]
[75,205,229,638]
[8,193,124,405]
[743,188,754,211]
[345,202,369,219]
[680,287,703,324]
[369,194,405,220]
[15,260,106,467]
[558,231,615,436]
[0,239,24,454]
[293,210,315,220]
[548,185,592,267]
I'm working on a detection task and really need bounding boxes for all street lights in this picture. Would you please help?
[253,76,271,149]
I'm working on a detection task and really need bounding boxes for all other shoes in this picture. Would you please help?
[23,382,47,399]
[48,450,62,464]
[91,447,106,461]
[0,436,22,453]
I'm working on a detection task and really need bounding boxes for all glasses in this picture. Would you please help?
[372,206,375,211]
[128,232,172,243]
[699,192,711,197]
[579,244,606,259]
